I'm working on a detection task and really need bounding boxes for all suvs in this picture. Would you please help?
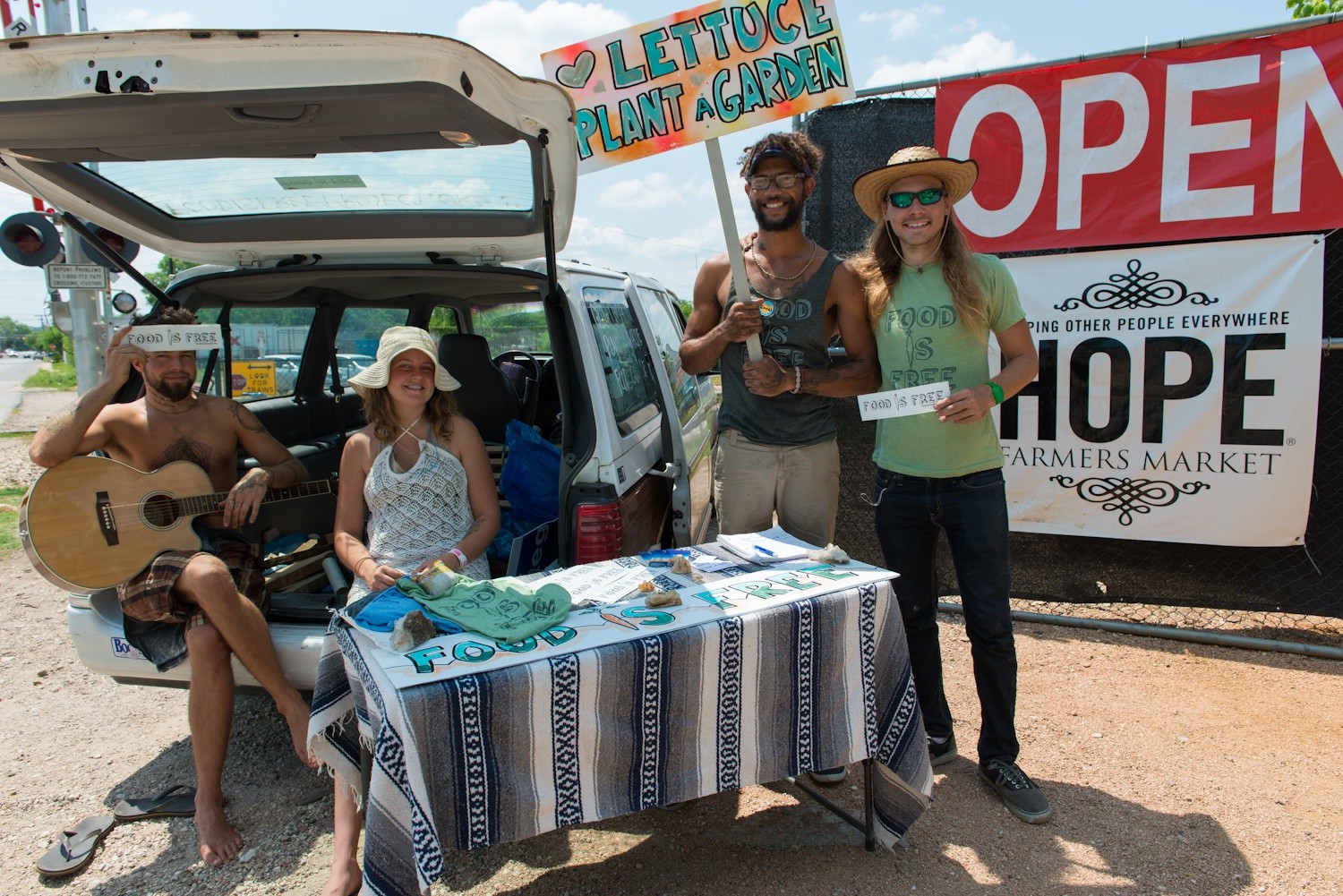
[0,28,722,701]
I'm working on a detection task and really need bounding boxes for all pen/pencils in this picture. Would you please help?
[752,543,778,557]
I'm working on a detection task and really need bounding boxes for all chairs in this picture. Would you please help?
[438,334,517,444]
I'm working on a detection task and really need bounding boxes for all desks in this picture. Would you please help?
[306,531,933,896]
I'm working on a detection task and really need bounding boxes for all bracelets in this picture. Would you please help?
[352,556,376,578]
[984,381,1004,404]
[788,365,801,394]
[447,546,470,570]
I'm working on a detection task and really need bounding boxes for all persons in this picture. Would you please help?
[322,322,504,896]
[678,129,882,549]
[26,307,327,868]
[849,143,1053,826]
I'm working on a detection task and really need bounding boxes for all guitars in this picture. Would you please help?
[19,455,340,595]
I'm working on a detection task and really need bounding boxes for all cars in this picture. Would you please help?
[4,348,43,360]
[258,354,348,395]
[335,353,377,378]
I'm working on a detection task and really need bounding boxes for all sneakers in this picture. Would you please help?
[978,758,1051,824]
[924,731,958,766]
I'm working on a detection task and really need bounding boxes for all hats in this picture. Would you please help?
[852,145,979,225]
[347,326,460,398]
[748,141,811,175]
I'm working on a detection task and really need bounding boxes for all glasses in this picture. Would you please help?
[885,187,944,208]
[745,172,806,189]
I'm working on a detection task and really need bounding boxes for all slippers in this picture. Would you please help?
[113,785,228,820]
[37,816,114,876]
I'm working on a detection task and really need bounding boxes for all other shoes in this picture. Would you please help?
[807,766,845,782]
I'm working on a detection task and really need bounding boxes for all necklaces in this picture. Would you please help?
[749,233,817,281]
